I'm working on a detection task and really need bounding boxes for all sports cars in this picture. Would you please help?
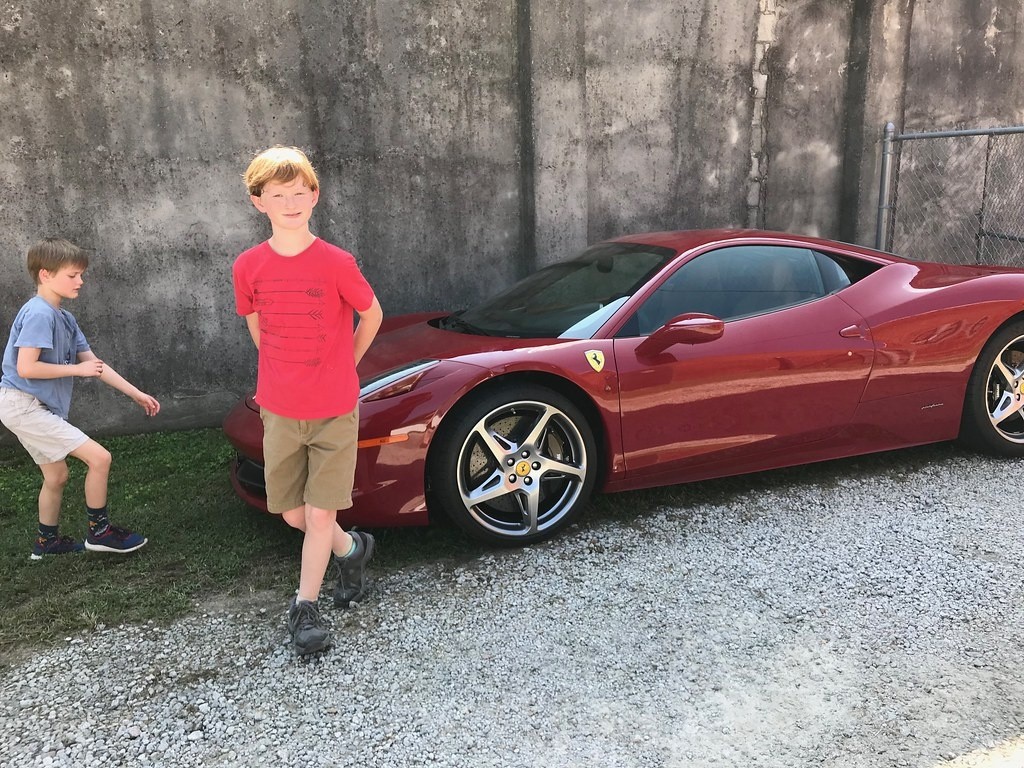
[220,227,1024,549]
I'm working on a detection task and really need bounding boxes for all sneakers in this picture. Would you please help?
[327,526,375,608]
[30,535,85,560]
[288,596,329,654]
[84,523,148,553]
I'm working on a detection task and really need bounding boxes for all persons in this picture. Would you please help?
[232,146,382,654]
[0,239,160,559]
